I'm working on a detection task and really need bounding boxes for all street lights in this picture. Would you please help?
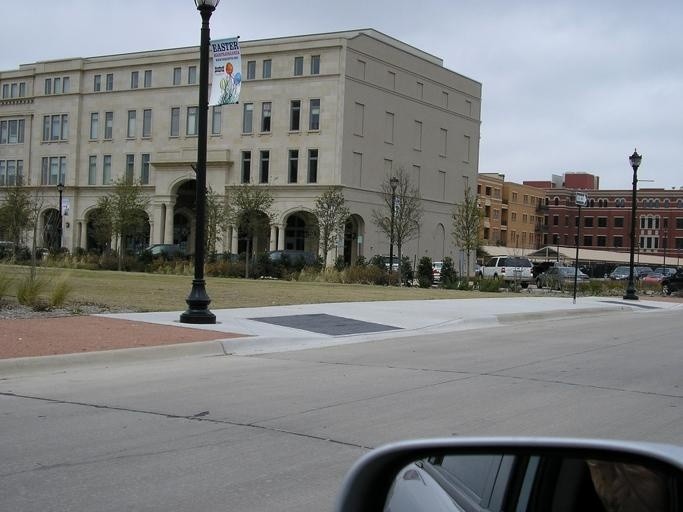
[55,182,65,249]
[623,148,642,300]
[180,0,217,322]
[387,176,399,286]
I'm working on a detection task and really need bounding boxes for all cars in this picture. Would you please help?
[535,268,590,291]
[431,261,445,282]
[611,266,682,296]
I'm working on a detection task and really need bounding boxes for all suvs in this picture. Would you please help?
[474,256,534,288]
[365,253,401,273]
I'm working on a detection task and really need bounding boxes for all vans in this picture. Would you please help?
[266,250,317,269]
[144,244,178,260]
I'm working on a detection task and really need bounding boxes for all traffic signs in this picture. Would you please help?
[576,193,588,207]
